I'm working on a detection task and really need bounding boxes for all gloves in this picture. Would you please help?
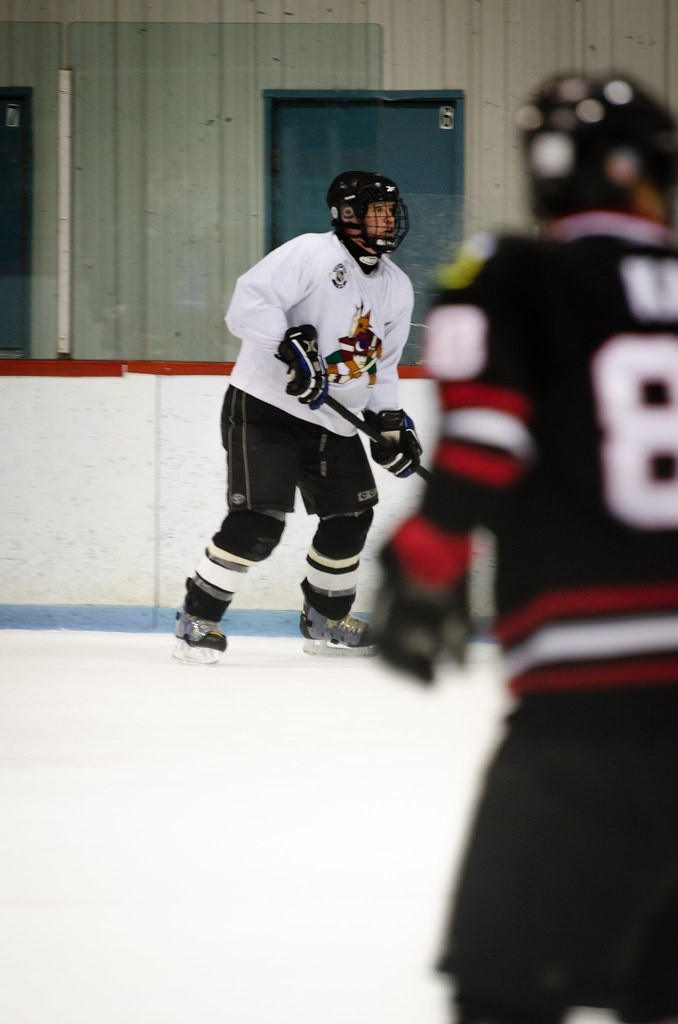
[369,540,475,680]
[362,407,422,479]
[274,323,329,410]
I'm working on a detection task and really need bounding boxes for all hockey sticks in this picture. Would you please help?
[273,351,434,486]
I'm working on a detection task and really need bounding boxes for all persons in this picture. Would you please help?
[174,170,422,665]
[377,69,678,1024]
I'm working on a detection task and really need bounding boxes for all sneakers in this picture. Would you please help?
[170,605,228,663]
[299,597,382,656]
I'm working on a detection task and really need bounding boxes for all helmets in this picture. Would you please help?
[326,170,410,253]
[521,67,678,218]
